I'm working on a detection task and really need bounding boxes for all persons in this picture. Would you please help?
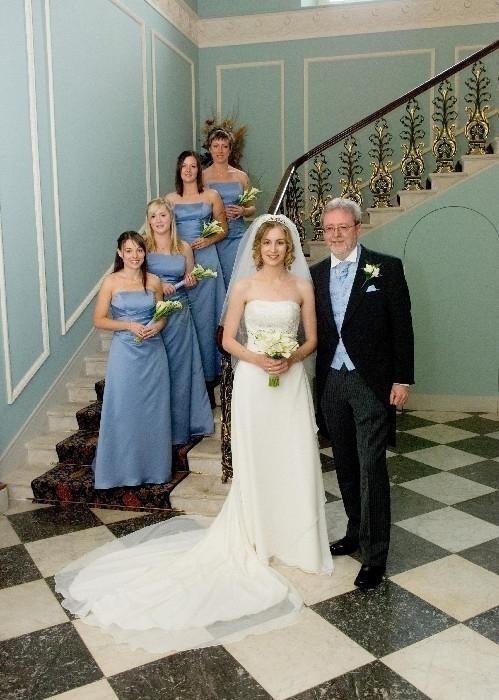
[54,213,334,653]
[201,129,256,289]
[93,232,173,490]
[140,198,215,445]
[310,198,415,589]
[166,151,227,383]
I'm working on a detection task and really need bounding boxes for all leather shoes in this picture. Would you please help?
[330,534,359,556]
[354,559,386,588]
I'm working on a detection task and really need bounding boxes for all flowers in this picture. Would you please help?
[357,263,381,293]
[199,217,224,240]
[171,264,221,290]
[233,184,263,209]
[133,299,184,342]
[265,334,298,387]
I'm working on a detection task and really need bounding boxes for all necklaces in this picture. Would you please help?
[156,240,171,250]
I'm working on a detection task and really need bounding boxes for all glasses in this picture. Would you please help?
[322,222,358,233]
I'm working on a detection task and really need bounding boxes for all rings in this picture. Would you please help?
[403,400,406,404]
[268,367,271,372]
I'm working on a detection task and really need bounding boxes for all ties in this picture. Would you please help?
[338,262,351,286]
